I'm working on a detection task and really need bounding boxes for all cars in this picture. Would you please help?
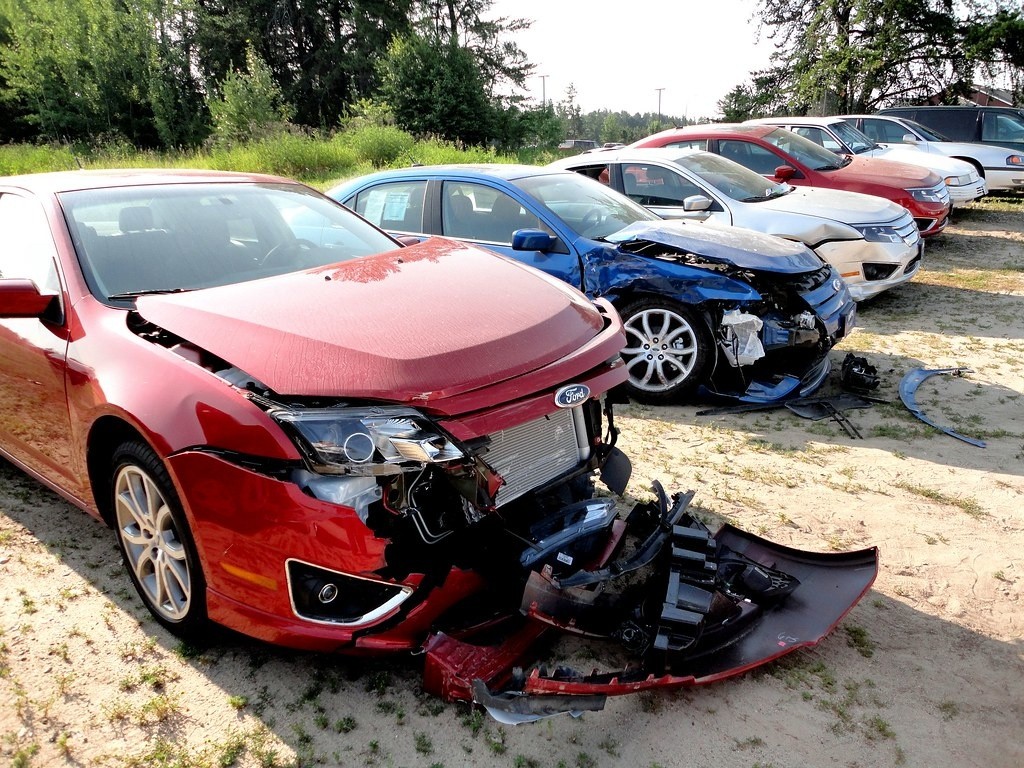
[602,124,954,237]
[0,169,630,654]
[539,144,925,306]
[727,117,990,202]
[301,162,860,411]
[773,115,1024,193]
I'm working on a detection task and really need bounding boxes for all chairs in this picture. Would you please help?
[176,204,257,286]
[626,173,644,198]
[491,194,522,244]
[450,194,488,237]
[404,188,424,231]
[118,205,178,289]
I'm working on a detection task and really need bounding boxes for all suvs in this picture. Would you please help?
[862,106,1024,151]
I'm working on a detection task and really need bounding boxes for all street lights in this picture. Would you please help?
[538,75,549,110]
[655,88,664,123]
